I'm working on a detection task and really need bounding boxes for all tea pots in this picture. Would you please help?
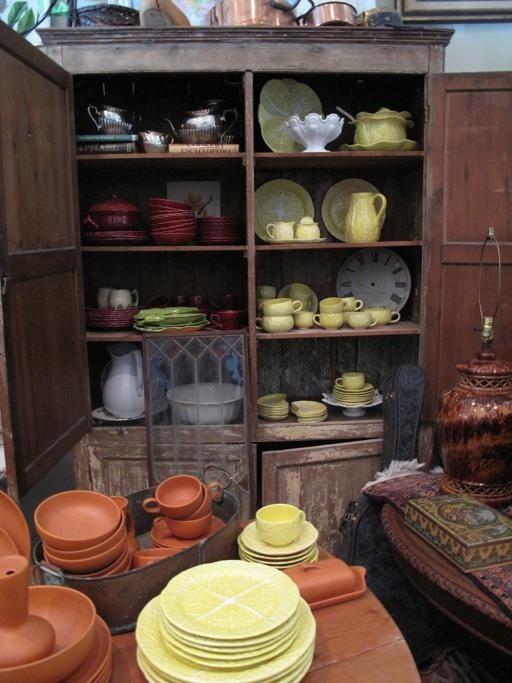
[344,192,387,243]
[164,108,238,144]
[137,129,174,152]
[88,105,133,135]
[100,343,146,418]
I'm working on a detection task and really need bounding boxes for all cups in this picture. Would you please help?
[318,298,349,313]
[297,216,322,240]
[151,512,214,541]
[261,297,303,316]
[145,295,170,308]
[312,313,346,331]
[340,297,364,310]
[133,548,182,568]
[98,287,115,308]
[265,221,296,242]
[142,475,204,519]
[211,310,242,330]
[346,312,377,330]
[257,297,273,312]
[255,503,306,547]
[294,311,315,330]
[257,286,277,297]
[368,310,401,326]
[254,316,295,333]
[109,288,139,307]
[335,372,364,389]
[184,481,222,520]
[213,294,238,309]
[185,294,209,308]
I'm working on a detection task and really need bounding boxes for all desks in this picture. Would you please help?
[380,501,512,661]
[0,520,425,683]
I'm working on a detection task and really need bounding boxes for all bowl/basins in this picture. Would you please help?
[38,528,129,573]
[42,509,125,560]
[283,111,344,153]
[167,383,244,426]
[33,489,129,553]
[76,533,139,580]
[1,586,97,683]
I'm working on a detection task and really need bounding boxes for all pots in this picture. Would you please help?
[84,194,138,230]
[349,106,415,144]
[300,1,366,27]
[204,0,317,28]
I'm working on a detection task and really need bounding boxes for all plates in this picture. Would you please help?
[91,403,171,422]
[252,179,315,244]
[135,594,317,683]
[54,614,115,683]
[339,140,419,149]
[320,387,385,417]
[277,284,318,312]
[84,230,145,244]
[290,400,329,424]
[258,392,290,421]
[332,384,376,408]
[320,178,386,244]
[0,490,31,560]
[197,217,240,244]
[264,236,328,243]
[85,306,139,331]
[237,521,319,571]
[156,559,302,673]
[131,306,211,334]
[150,516,226,550]
[0,529,19,559]
[147,198,198,244]
[257,78,323,152]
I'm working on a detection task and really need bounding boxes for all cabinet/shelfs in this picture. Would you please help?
[0,17,512,568]
